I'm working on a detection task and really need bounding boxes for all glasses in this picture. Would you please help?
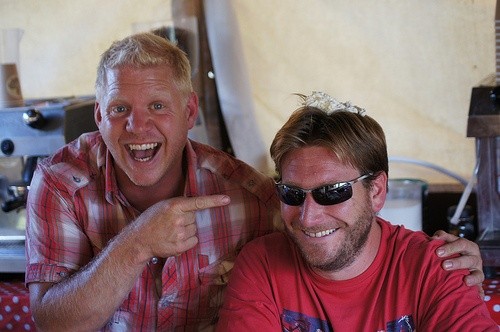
[275,172,372,206]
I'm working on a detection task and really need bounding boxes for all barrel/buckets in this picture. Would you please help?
[377,177,428,233]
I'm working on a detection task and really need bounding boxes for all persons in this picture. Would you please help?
[216,105,500,332]
[25,34,486,332]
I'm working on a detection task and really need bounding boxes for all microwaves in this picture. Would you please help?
[0,98,101,272]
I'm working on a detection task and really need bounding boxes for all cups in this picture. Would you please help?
[447,206,475,244]
[0,63,23,107]
